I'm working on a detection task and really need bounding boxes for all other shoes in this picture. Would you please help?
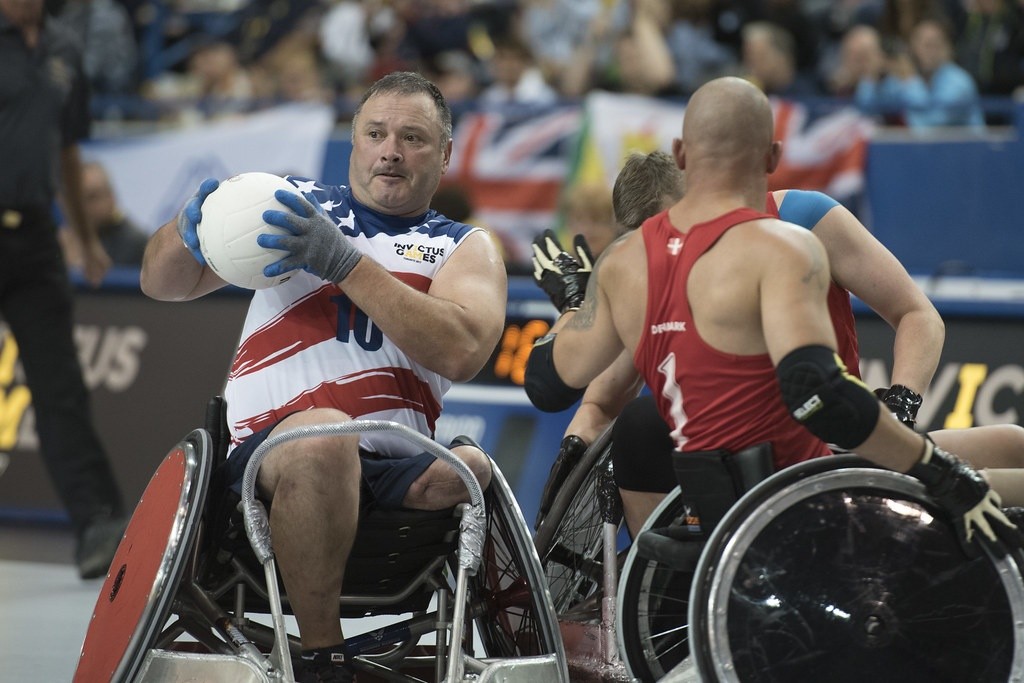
[302,663,357,683]
[74,509,129,577]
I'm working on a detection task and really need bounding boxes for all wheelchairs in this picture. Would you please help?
[523,392,1023,683]
[73,393,571,683]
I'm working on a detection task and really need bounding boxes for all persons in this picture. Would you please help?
[0,1,1024,681]
[138,70,509,682]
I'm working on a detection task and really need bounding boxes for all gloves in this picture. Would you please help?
[531,228,596,319]
[906,433,1023,559]
[257,189,363,285]
[176,177,219,265]
[872,384,923,430]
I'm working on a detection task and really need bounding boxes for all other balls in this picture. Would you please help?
[193,170,312,294]
